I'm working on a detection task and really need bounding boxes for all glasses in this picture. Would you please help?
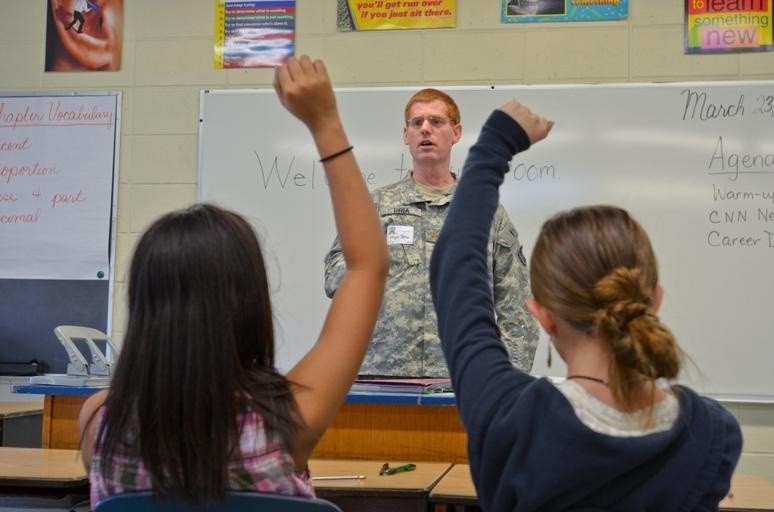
[406,115,455,129]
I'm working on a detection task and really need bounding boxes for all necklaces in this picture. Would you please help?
[565,370,648,390]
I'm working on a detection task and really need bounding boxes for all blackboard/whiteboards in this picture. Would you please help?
[0,89,124,385]
[196,80,774,404]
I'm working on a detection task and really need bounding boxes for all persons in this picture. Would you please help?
[318,86,539,384]
[431,97,742,512]
[76,53,392,512]
[65,0,94,33]
[46,0,123,72]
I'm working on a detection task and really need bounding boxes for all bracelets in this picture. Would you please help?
[319,143,357,165]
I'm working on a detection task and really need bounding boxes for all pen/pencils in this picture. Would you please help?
[379,464,416,475]
[311,476,366,480]
